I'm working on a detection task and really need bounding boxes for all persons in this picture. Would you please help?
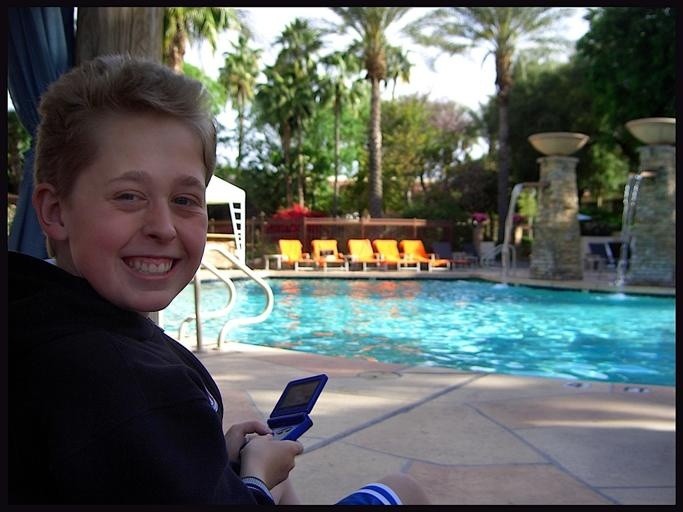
[1,54,434,506]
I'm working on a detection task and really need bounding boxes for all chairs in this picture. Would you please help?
[265,239,481,274]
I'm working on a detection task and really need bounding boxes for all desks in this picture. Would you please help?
[200,234,236,269]
